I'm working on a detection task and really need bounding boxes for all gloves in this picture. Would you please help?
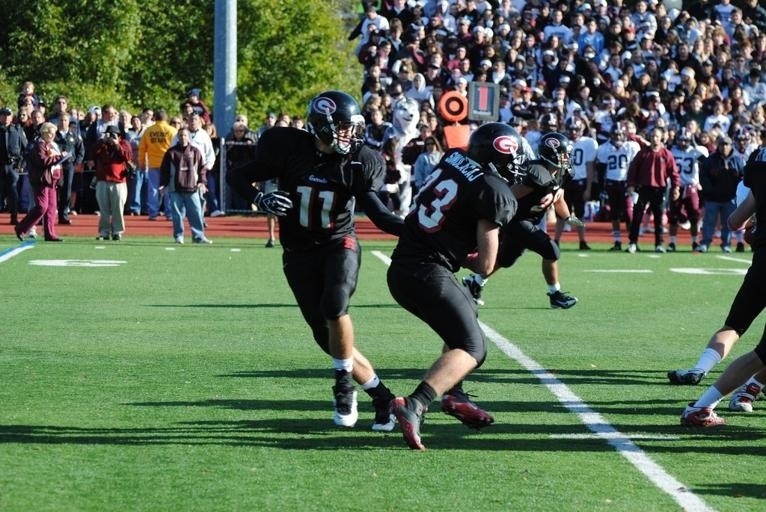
[255,193,294,215]
[566,217,586,229]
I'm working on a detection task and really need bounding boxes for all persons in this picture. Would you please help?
[347,0,504,121]
[0,108,27,225]
[700,138,742,254]
[17,122,77,241]
[463,131,586,310]
[500,1,766,123]
[680,325,766,428]
[386,122,530,450]
[626,129,680,256]
[225,91,405,431]
[415,136,444,195]
[381,136,401,215]
[15,80,209,127]
[227,108,305,145]
[173,112,216,229]
[667,127,706,252]
[729,128,761,252]
[48,113,85,226]
[161,128,214,245]
[138,109,180,221]
[667,141,766,387]
[365,111,391,150]
[550,115,600,250]
[90,126,135,241]
[597,122,640,252]
[406,124,440,207]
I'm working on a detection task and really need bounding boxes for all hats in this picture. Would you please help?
[521,87,532,95]
[717,135,732,145]
[88,106,102,114]
[102,125,123,135]
[1,107,12,115]
[232,122,247,130]
[185,89,200,97]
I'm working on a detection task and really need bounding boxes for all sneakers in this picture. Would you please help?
[610,241,621,252]
[692,242,700,251]
[578,239,589,251]
[265,238,275,248]
[45,235,64,243]
[198,237,214,245]
[699,245,707,252]
[57,220,73,225]
[373,390,400,430]
[461,273,483,306]
[14,225,25,244]
[667,242,676,251]
[563,224,570,232]
[730,386,755,412]
[656,243,666,253]
[549,291,578,311]
[626,243,638,252]
[392,396,426,451]
[638,226,645,235]
[643,226,655,233]
[666,367,701,387]
[96,233,109,240]
[176,234,185,245]
[331,383,358,431]
[680,400,715,427]
[441,392,494,429]
[28,227,38,238]
[721,245,732,252]
[111,235,122,240]
[210,208,225,216]
[737,241,744,254]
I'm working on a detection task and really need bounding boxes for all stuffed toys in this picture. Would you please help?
[384,98,421,216]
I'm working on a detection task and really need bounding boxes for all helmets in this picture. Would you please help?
[468,123,527,187]
[734,129,753,143]
[613,121,629,137]
[308,92,366,153]
[567,116,582,131]
[677,126,692,139]
[538,132,571,172]
[541,113,559,126]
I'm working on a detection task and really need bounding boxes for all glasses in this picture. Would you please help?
[425,141,437,148]
[19,113,26,117]
[56,100,67,107]
[171,120,182,125]
